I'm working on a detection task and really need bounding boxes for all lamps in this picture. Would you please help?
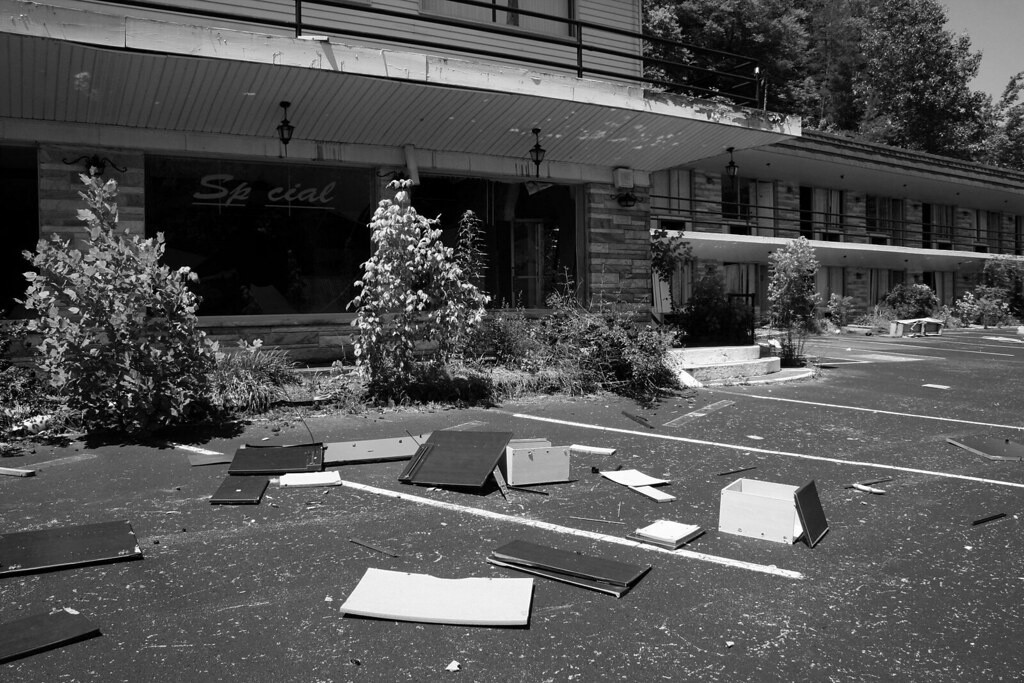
[277,99,295,158]
[726,146,738,187]
[529,127,546,178]
